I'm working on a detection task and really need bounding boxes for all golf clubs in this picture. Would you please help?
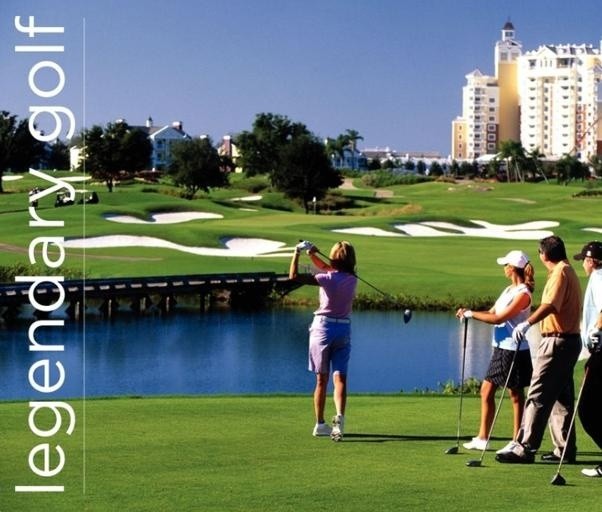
[297,238,411,325]
[551,350,596,485]
[466,341,521,466]
[445,316,468,456]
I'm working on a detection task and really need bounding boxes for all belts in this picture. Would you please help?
[542,332,565,338]
[322,317,351,325]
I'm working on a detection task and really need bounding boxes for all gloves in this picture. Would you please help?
[460,310,473,323]
[297,240,313,251]
[587,328,602,354]
[512,321,531,344]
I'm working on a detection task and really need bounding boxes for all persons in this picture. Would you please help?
[574,240,602,477]
[289,240,358,441]
[455,250,536,451]
[29,188,40,210]
[496,235,577,463]
[54,190,99,207]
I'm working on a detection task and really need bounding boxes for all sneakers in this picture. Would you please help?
[494,440,537,464]
[462,437,490,451]
[581,467,602,477]
[330,415,344,442]
[541,452,561,461]
[312,423,332,437]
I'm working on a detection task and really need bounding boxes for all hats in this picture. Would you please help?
[573,240,601,260]
[496,250,529,269]
